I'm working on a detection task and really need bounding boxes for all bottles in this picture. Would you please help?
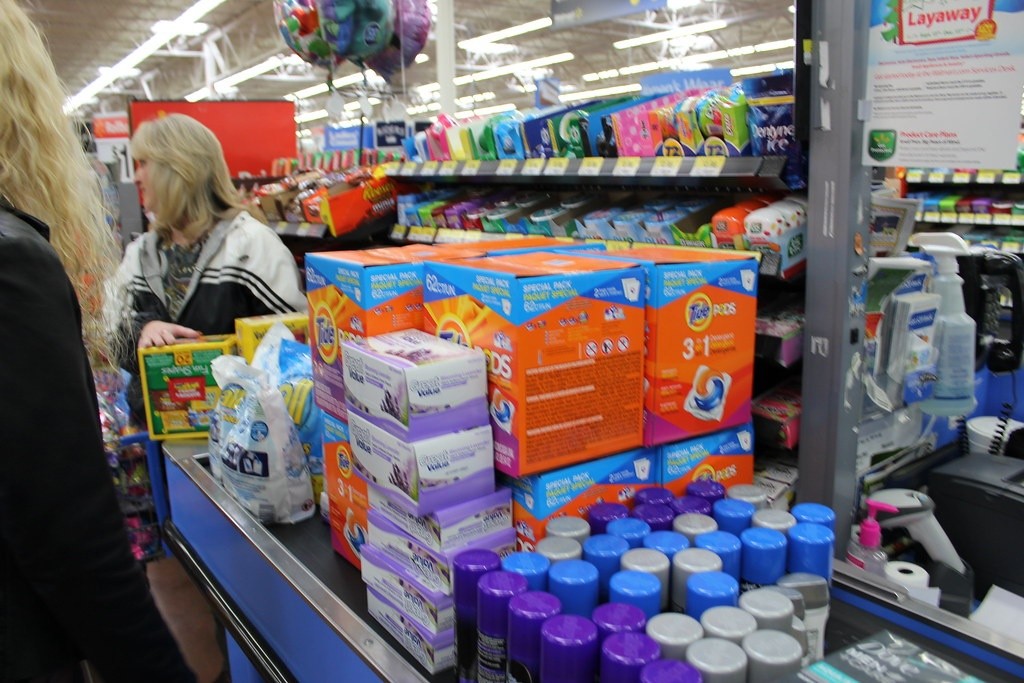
[920,262,976,416]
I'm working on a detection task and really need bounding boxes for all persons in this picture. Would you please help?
[99,113,307,683]
[1,1,205,683]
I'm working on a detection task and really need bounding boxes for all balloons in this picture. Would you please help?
[274,0,432,86]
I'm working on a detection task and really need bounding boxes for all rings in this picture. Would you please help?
[161,329,168,335]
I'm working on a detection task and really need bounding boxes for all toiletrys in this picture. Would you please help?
[845,500,899,577]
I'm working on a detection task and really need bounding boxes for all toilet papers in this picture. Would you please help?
[881,559,930,599]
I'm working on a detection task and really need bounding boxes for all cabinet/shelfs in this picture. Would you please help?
[373,156,801,468]
[901,167,1024,261]
[227,165,374,275]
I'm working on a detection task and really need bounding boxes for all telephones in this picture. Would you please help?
[956,250,1024,373]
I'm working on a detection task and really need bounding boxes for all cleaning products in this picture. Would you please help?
[905,234,976,413]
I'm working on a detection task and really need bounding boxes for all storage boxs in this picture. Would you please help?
[132,68,805,675]
